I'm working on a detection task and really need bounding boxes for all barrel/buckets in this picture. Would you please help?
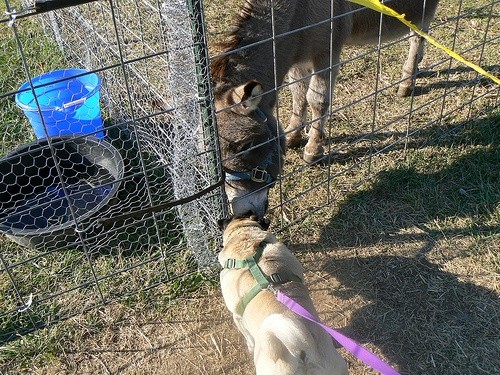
[15,68,105,141]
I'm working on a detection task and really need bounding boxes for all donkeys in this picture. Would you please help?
[213,0,442,217]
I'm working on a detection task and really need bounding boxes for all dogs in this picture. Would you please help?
[215,215,348,374]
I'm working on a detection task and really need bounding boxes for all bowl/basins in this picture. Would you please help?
[0,132,124,249]
